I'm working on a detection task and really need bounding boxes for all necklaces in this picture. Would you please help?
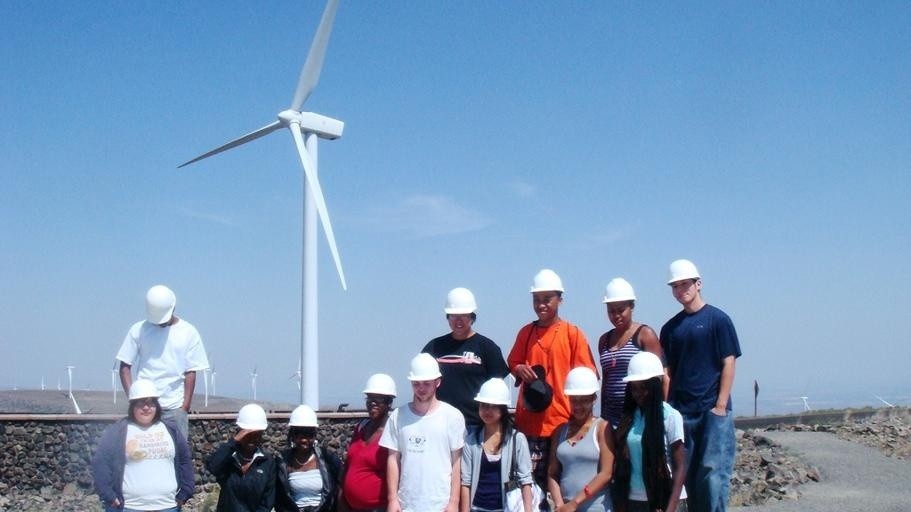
[238,449,253,462]
[536,320,561,354]
[294,452,315,466]
[568,422,589,447]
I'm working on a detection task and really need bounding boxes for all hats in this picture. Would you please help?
[522,364,554,413]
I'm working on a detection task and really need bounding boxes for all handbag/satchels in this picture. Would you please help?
[502,435,543,512]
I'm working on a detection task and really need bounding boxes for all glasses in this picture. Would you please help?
[366,396,384,406]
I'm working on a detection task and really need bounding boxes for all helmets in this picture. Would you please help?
[236,403,269,431]
[473,377,513,407]
[444,286,478,317]
[129,377,161,401]
[530,269,564,293]
[562,367,600,397]
[665,259,699,284]
[622,350,664,383]
[602,278,637,305]
[361,374,397,398]
[144,285,176,326]
[285,404,319,428]
[408,351,442,382]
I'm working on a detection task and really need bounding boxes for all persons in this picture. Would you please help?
[117,286,208,444]
[93,380,196,511]
[547,367,615,511]
[460,377,534,511]
[659,259,741,512]
[599,278,669,421]
[341,374,398,511]
[273,404,342,511]
[421,288,509,435]
[203,404,278,511]
[379,351,466,512]
[615,351,687,511]
[507,268,602,511]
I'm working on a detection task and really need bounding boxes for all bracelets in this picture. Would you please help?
[584,484,593,501]
